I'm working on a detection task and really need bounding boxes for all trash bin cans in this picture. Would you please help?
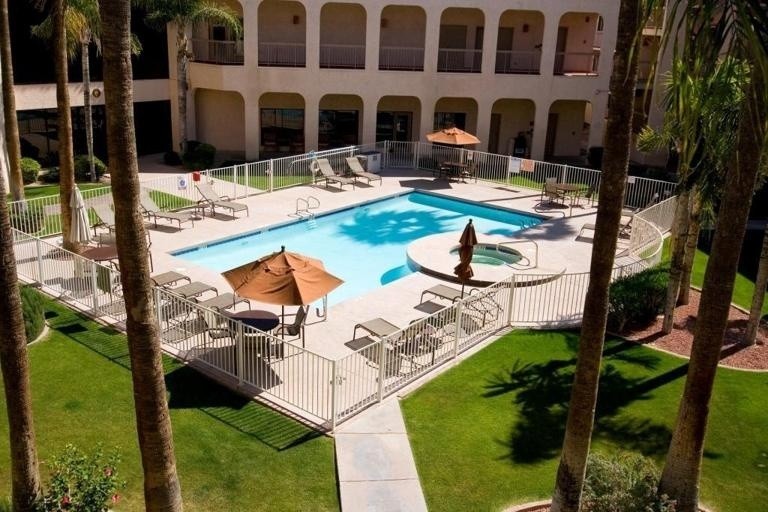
[356,151,382,174]
[185,140,216,171]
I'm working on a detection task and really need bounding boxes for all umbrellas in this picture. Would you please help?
[222,244,346,359]
[426,125,480,165]
[454,218,479,298]
[69,183,93,245]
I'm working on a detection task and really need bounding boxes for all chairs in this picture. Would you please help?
[315,156,383,192]
[352,317,424,361]
[419,282,500,326]
[434,161,478,183]
[150,270,309,361]
[576,191,659,242]
[91,182,249,244]
[540,177,596,211]
